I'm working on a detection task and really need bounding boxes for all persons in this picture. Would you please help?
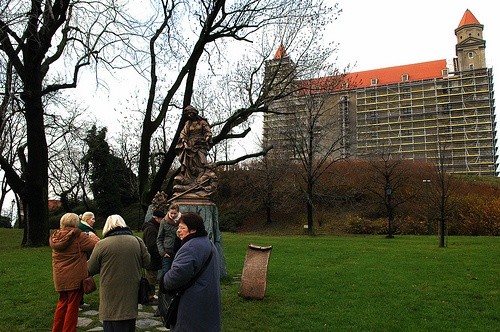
[174,105,217,185]
[161,212,221,332]
[78,211,98,304]
[87,214,150,332]
[49,213,98,332]
[141,205,167,306]
[154,204,186,317]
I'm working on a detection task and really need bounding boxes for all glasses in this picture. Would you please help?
[177,226,186,231]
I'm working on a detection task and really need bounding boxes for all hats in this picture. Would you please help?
[152,210,166,218]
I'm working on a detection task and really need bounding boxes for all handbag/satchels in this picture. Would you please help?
[158,289,180,329]
[83,276,97,294]
[138,277,151,305]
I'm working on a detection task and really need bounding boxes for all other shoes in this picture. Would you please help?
[81,303,91,306]
[138,304,143,310]
[78,308,83,311]
[153,294,158,299]
[155,290,159,292]
